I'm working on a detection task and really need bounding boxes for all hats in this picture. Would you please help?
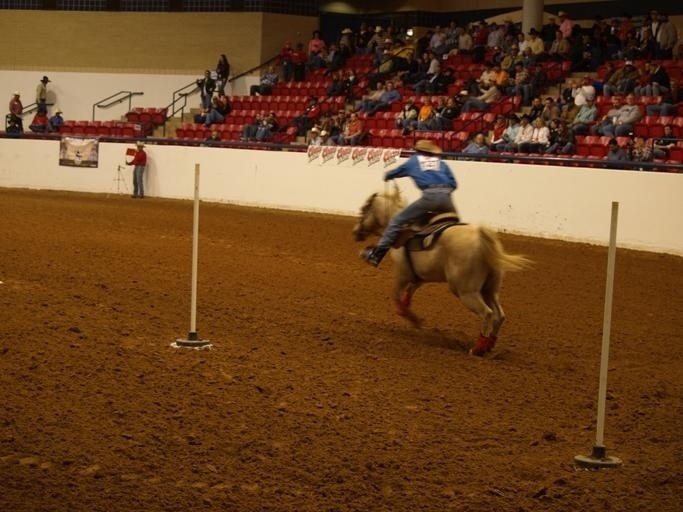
[493,111,532,120]
[584,95,595,103]
[477,12,569,71]
[624,60,632,67]
[374,26,384,32]
[342,28,353,34]
[408,140,443,155]
[135,141,145,147]
[41,76,50,82]
[14,91,20,96]
[55,108,62,114]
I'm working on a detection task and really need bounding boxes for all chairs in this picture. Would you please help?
[59,107,167,142]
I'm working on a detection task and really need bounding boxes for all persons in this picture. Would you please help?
[7,118,18,133]
[359,139,457,267]
[8,91,24,133]
[125,141,146,198]
[48,108,63,130]
[35,76,51,113]
[195,11,683,173]
[28,108,48,133]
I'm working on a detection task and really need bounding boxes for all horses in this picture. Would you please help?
[350,189,537,359]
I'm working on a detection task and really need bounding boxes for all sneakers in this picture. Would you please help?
[131,195,143,198]
[359,246,387,265]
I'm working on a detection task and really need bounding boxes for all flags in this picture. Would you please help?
[59,136,98,168]
